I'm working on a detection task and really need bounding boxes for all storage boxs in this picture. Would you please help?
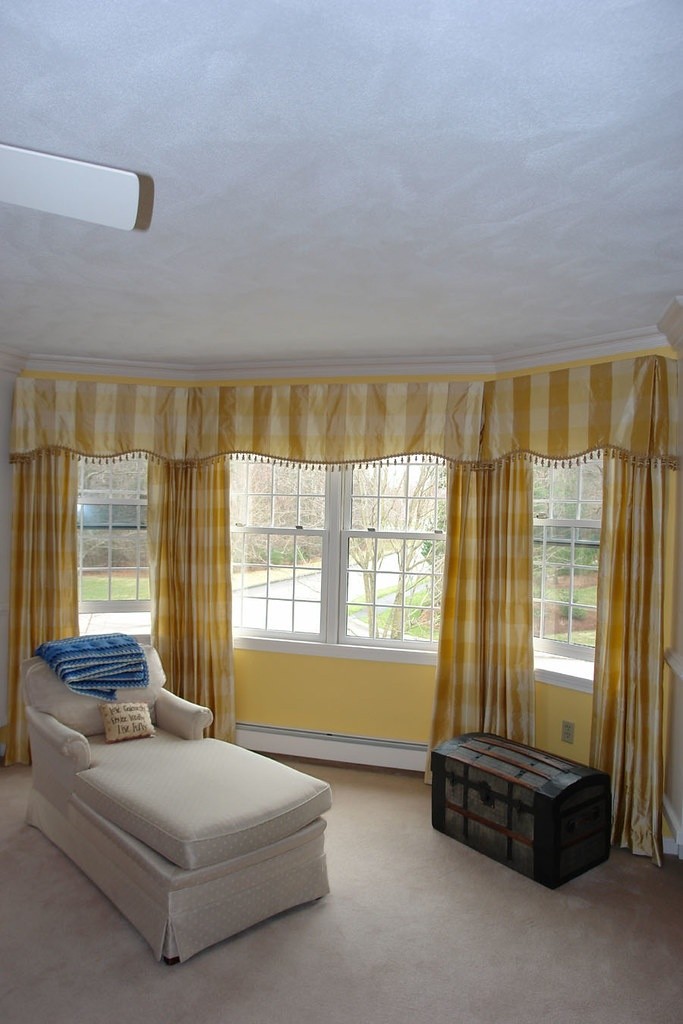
[431,732,611,889]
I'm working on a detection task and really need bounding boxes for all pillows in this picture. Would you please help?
[97,702,156,743]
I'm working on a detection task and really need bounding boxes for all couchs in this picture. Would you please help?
[18,643,334,966]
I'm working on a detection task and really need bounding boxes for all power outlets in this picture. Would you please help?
[561,720,575,744]
